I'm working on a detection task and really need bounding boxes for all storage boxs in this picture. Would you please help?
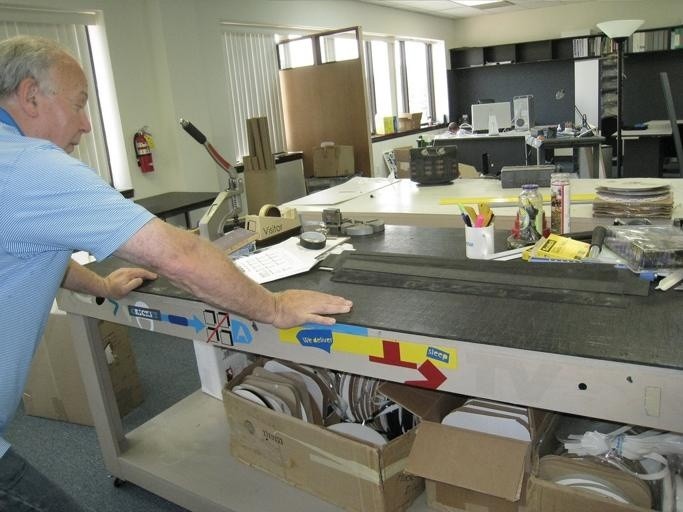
[223,359,425,511]
[524,416,654,511]
[376,382,554,512]
[20,297,144,424]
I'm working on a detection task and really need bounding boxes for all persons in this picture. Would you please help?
[0,36,353,512]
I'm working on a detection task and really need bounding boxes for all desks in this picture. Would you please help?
[432,120,683,178]
[133,191,218,230]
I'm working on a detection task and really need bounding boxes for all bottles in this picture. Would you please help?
[488,115,499,136]
[550,171,571,237]
[518,183,544,241]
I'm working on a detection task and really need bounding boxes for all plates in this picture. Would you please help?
[233,357,397,447]
[596,177,671,196]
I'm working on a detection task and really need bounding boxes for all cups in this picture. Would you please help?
[464,222,495,262]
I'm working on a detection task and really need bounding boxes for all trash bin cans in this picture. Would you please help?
[573,143,613,179]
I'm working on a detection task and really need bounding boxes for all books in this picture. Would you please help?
[572,28,683,58]
[523,233,590,262]
[212,228,260,255]
[470,60,511,67]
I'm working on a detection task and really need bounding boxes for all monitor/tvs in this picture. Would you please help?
[471,102,512,136]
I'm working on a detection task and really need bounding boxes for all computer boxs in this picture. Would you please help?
[513,95,535,132]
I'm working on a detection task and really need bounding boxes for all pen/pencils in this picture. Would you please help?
[459,202,495,227]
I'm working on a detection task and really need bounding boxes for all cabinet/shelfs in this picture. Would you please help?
[447,25,683,70]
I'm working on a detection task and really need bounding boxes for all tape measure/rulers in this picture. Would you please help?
[439,193,598,207]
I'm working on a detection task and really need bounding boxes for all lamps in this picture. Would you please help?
[597,19,645,176]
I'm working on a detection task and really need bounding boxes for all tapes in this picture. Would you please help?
[300,231,327,250]
[259,204,283,217]
